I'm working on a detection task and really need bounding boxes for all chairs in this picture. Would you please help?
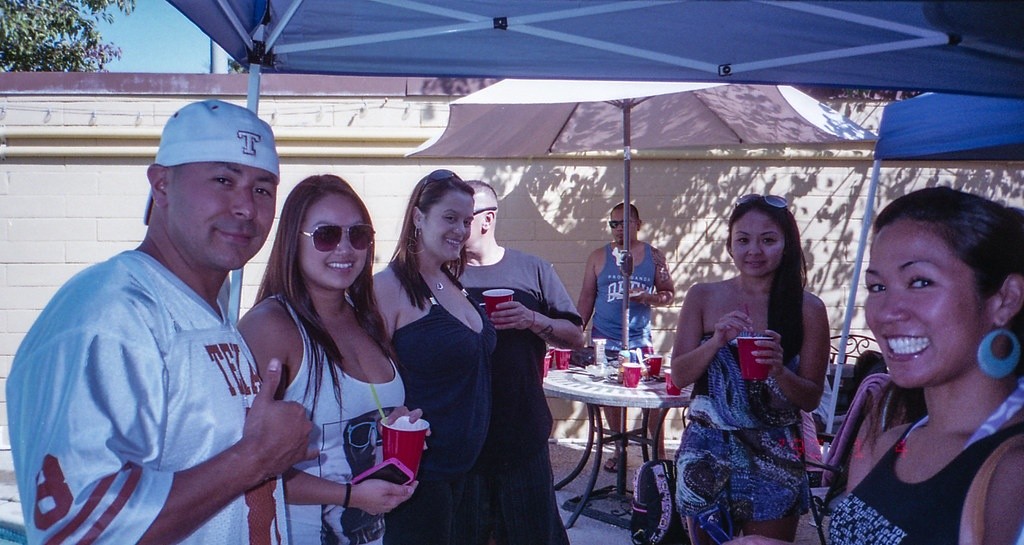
[804,391,870,545]
[823,335,888,434]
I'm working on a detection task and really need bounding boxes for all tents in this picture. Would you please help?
[163,0,1024,435]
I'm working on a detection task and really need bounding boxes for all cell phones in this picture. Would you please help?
[352,458,414,486]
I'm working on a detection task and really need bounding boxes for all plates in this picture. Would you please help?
[607,290,644,299]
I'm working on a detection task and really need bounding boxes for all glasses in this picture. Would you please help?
[411,169,463,206]
[734,194,787,209]
[609,219,638,229]
[297,224,375,252]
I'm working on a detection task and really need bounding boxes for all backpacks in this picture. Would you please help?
[630,460,674,545]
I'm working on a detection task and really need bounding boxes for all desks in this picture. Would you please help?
[542,362,694,528]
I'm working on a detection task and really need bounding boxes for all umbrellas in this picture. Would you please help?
[404,79,886,357]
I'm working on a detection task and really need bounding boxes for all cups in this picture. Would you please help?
[621,362,642,388]
[646,355,663,378]
[380,416,429,482]
[482,289,514,320]
[543,347,571,378]
[737,336,774,380]
[664,369,681,395]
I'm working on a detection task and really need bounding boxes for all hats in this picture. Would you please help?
[143,101,282,225]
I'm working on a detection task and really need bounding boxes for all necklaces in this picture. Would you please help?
[422,266,447,292]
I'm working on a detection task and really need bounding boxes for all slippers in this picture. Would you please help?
[604,457,618,473]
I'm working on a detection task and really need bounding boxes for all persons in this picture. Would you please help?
[829,186,1024,545]
[574,203,676,474]
[670,194,830,545]
[6,101,321,545]
[441,180,585,545]
[351,171,497,545]
[236,175,430,545]
